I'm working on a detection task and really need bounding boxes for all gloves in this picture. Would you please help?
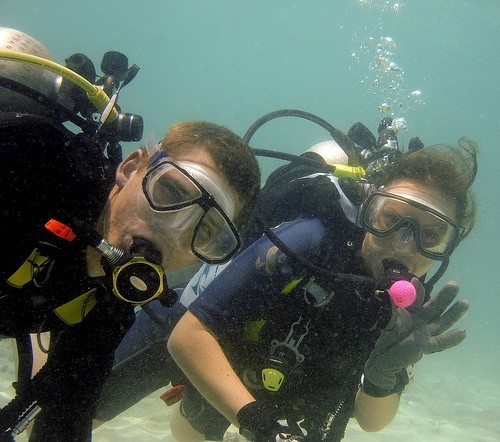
[364,276,469,392]
[237,397,322,442]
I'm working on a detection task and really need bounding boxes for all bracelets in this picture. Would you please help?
[357,371,405,398]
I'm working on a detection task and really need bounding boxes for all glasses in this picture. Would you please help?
[140,150,242,267]
[360,189,461,261]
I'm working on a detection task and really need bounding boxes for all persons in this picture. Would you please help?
[12,144,471,440]
[0,108,259,440]
